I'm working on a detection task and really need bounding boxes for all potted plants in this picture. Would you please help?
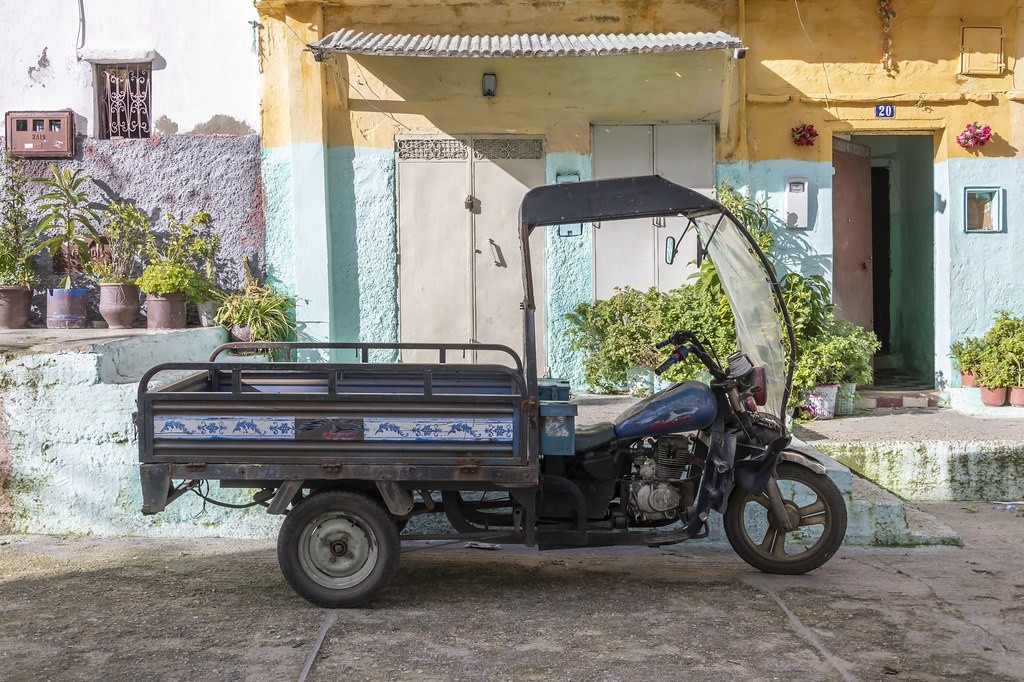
[23,163,102,331]
[563,181,883,423]
[0,149,36,329]
[136,212,220,329]
[947,307,1024,407]
[85,201,153,330]
[213,257,300,362]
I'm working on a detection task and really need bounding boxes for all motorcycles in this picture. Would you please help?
[132,174,849,610]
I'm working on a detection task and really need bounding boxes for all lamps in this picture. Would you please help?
[481,72,496,96]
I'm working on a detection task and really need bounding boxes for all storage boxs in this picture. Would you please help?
[539,400,577,456]
[537,378,571,400]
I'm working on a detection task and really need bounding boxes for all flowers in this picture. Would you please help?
[791,123,819,147]
[956,121,992,148]
